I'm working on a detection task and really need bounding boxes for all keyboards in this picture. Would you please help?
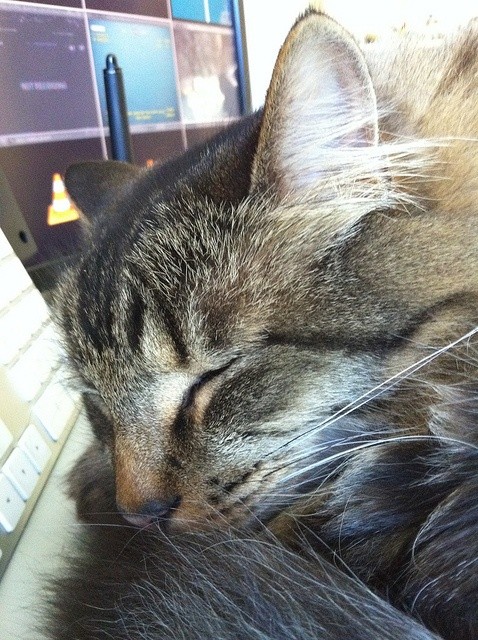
[1,229,83,587]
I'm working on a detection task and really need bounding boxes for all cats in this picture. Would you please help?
[28,2,476,638]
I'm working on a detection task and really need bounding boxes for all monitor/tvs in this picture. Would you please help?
[0,1,255,293]
